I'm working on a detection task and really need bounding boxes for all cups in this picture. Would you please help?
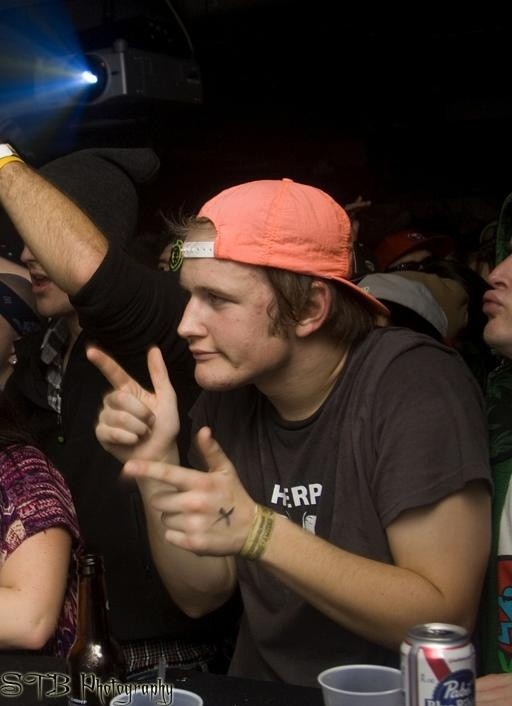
[109,687,204,706]
[318,665,403,706]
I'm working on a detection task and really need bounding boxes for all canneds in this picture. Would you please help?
[398,622,477,706]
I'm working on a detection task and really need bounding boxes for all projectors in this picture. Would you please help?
[31,47,203,114]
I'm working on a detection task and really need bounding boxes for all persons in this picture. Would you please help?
[2,142,510,706]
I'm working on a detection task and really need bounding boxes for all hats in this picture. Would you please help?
[180,178,469,343]
[37,147,160,239]
